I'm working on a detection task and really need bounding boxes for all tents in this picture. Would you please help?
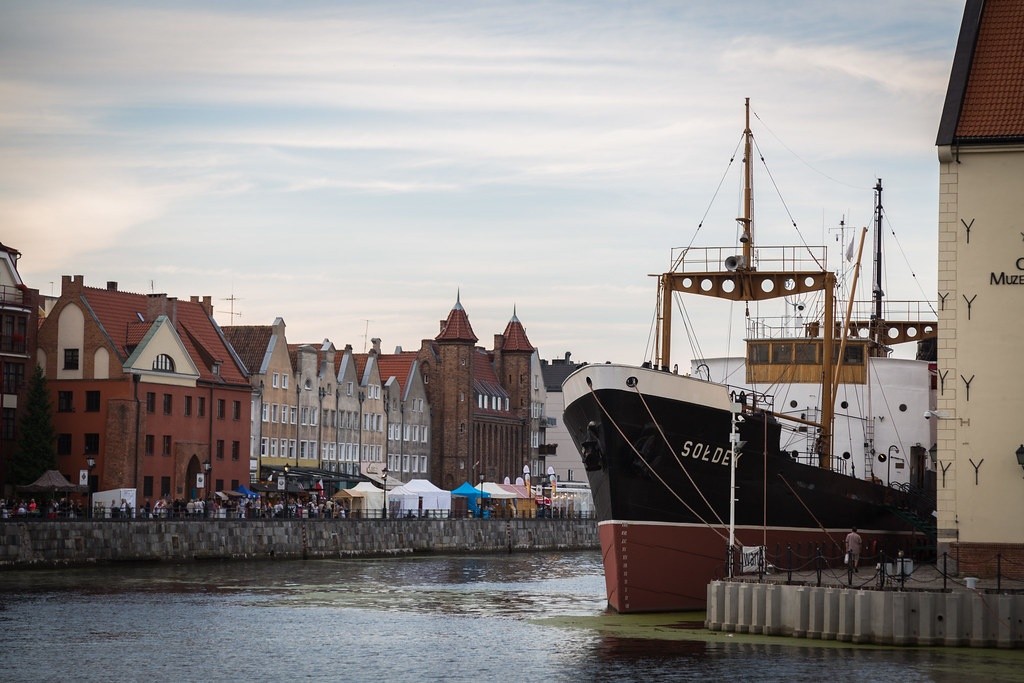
[452,482,491,519]
[352,482,390,518]
[474,482,517,509]
[388,486,419,517]
[405,479,451,518]
[233,486,260,500]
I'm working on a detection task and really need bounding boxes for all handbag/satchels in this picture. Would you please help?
[844,553,849,564]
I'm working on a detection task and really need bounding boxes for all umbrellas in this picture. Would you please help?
[16,470,87,502]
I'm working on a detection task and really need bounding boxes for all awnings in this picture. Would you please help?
[223,490,245,496]
[216,492,228,500]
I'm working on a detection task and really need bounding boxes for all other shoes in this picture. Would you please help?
[855,569,858,573]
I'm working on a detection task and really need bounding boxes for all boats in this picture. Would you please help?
[560,89,939,616]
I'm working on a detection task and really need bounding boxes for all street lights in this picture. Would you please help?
[381,467,389,518]
[86,454,95,518]
[283,461,291,516]
[479,470,485,516]
[201,458,212,516]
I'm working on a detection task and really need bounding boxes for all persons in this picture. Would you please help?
[0,497,339,519]
[846,527,862,572]
